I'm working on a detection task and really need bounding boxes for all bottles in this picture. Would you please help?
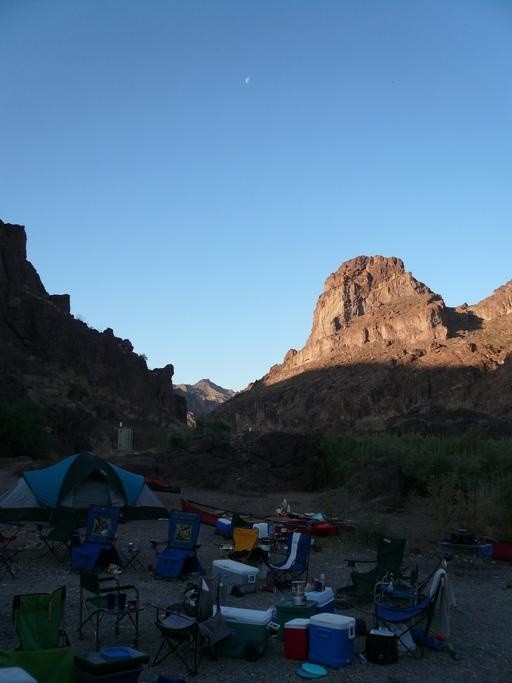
[219,574,232,605]
[287,570,326,605]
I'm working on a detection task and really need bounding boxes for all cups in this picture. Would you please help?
[107,592,128,609]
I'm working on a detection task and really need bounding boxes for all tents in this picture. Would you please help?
[0,451,166,522]
